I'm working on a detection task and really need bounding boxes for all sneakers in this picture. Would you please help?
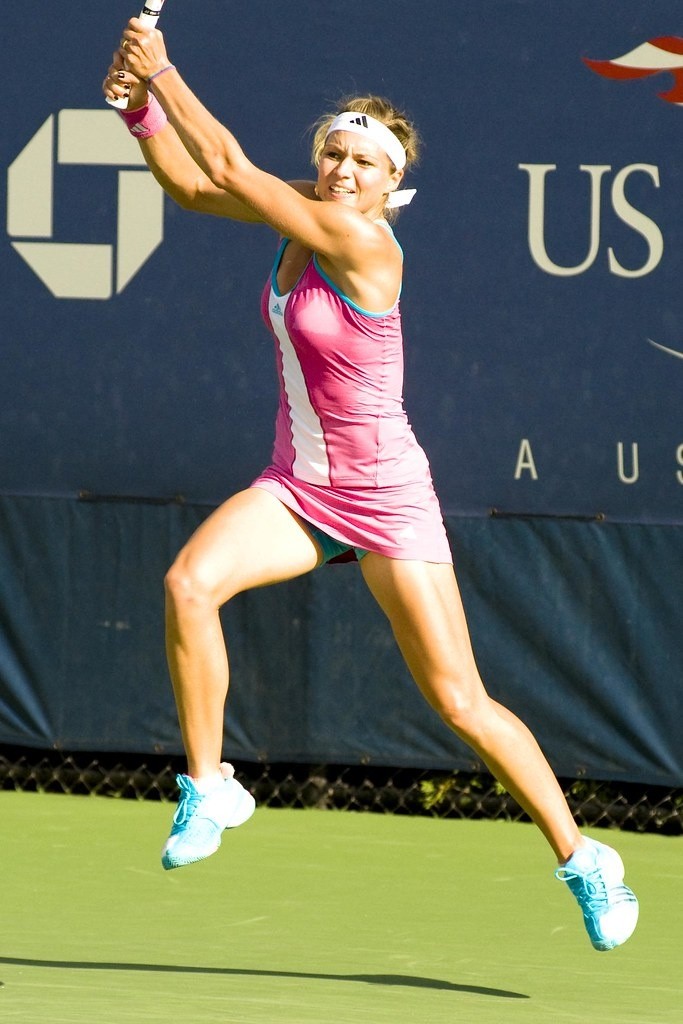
[161,761,256,869]
[554,835,638,950]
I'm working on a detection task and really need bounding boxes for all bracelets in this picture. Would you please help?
[120,90,167,138]
[147,65,177,91]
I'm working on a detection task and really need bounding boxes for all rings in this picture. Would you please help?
[123,40,128,49]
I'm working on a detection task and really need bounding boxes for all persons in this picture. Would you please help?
[103,17,639,950]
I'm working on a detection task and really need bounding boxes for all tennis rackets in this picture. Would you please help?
[106,0,164,110]
